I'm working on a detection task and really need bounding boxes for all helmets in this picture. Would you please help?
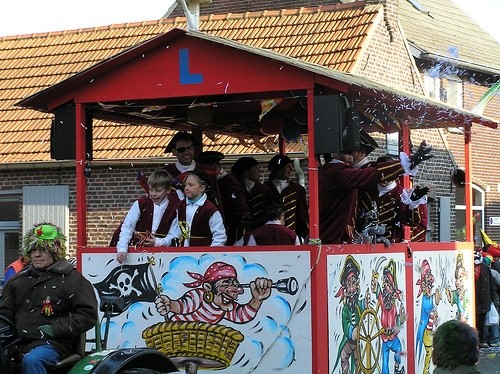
[474,251,483,265]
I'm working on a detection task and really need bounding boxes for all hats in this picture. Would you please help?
[163,131,201,154]
[20,222,66,262]
[231,157,259,176]
[198,151,224,163]
[268,155,294,171]
[360,129,379,149]
[481,252,493,265]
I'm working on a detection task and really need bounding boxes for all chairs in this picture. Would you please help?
[50,331,87,374]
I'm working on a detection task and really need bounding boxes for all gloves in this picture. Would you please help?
[37,325,54,338]
[410,184,430,201]
[408,140,432,170]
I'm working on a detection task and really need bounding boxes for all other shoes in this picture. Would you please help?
[480,342,500,347]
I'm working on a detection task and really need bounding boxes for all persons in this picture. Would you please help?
[136,170,227,250]
[0,220,99,374]
[453,229,500,349]
[130,123,438,246]
[431,320,484,374]
[115,170,183,263]
[5,251,31,288]
